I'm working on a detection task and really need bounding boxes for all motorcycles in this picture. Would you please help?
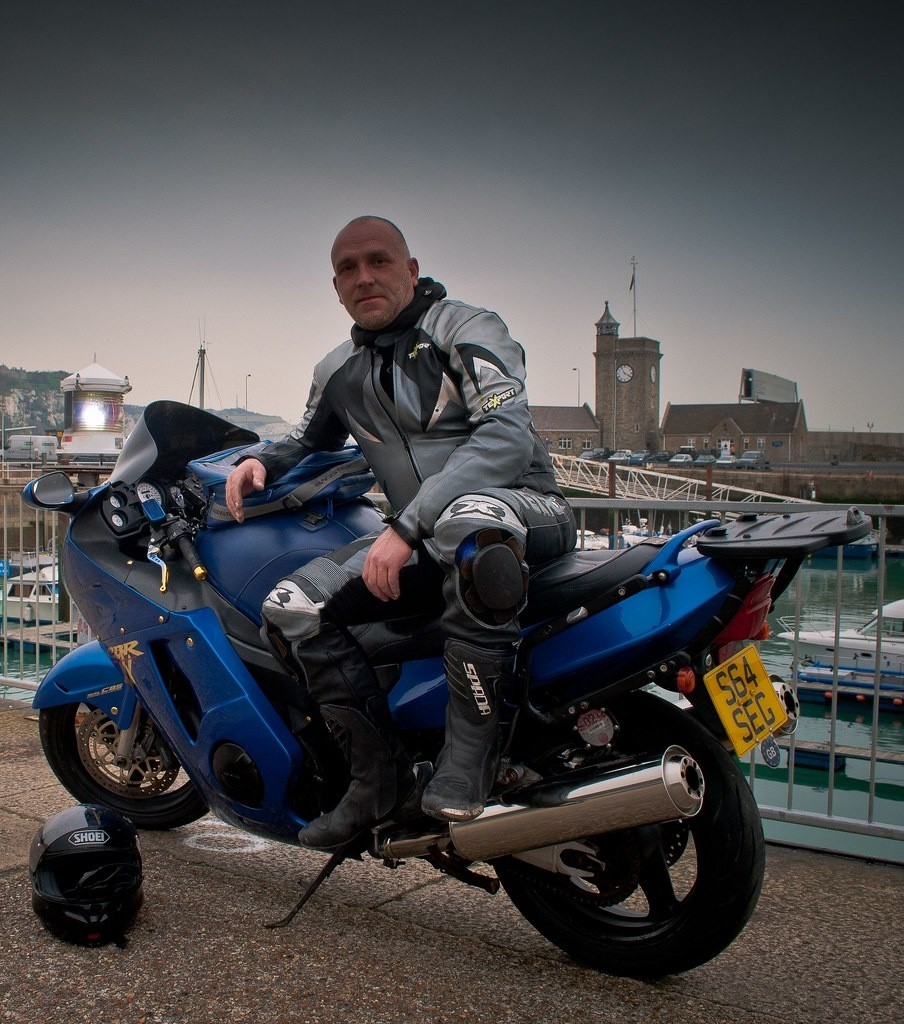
[22,403,873,983]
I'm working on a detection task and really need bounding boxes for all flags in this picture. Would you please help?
[629,274,634,290]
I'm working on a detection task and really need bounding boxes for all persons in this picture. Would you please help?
[225,215,577,850]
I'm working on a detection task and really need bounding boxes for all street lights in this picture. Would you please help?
[245,374,251,410]
[572,367,581,407]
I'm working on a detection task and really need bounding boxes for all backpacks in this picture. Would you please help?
[189,438,377,526]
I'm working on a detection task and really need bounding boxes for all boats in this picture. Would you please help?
[813,527,888,558]
[0,536,58,655]
[575,518,698,552]
[774,599,904,710]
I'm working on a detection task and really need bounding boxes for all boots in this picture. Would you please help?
[422,640,517,822]
[282,621,418,850]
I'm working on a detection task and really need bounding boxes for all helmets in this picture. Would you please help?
[28,803,142,949]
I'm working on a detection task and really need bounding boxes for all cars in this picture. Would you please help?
[617,449,633,455]
[608,453,629,464]
[630,450,655,466]
[716,456,736,468]
[576,450,601,464]
[693,455,716,467]
[668,454,693,467]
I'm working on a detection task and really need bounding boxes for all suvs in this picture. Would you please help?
[593,447,615,459]
[737,450,765,468]
[655,450,675,462]
[678,446,735,461]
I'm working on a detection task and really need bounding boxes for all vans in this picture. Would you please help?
[0,435,59,469]
